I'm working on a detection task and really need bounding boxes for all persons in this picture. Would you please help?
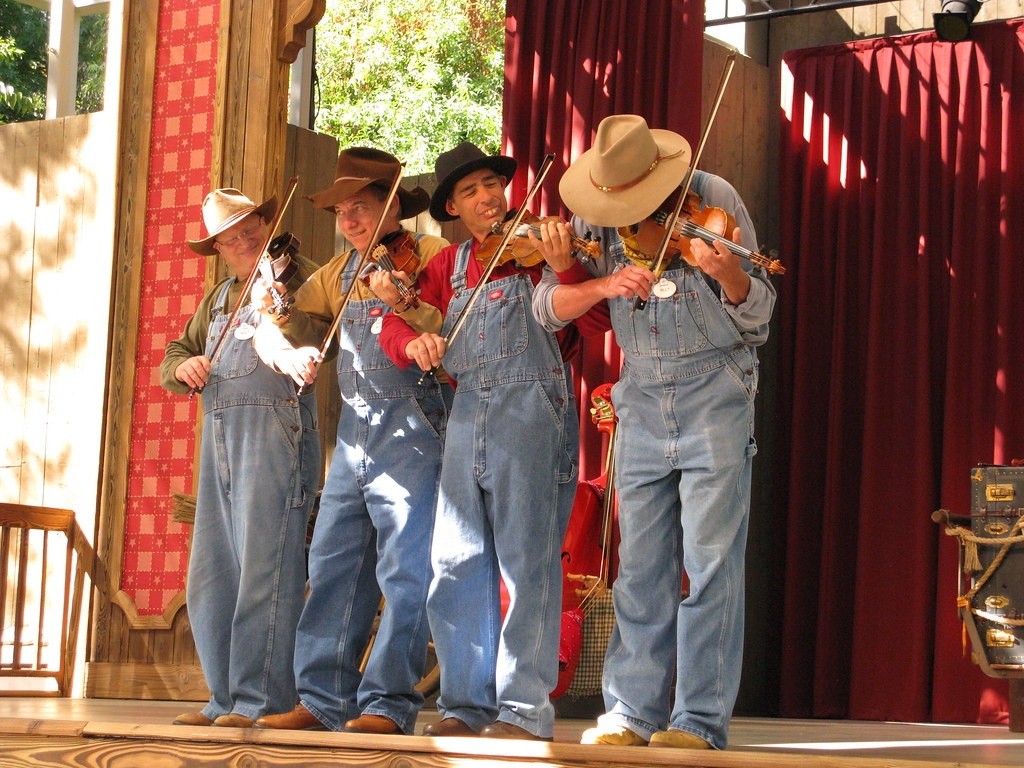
[252,147,454,736]
[160,188,341,728]
[532,114,777,750]
[379,142,614,743]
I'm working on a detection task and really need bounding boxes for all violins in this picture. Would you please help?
[635,185,787,276]
[259,232,302,323]
[358,230,421,311]
[476,208,603,268]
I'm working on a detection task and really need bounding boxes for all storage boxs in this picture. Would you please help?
[969,468,1024,665]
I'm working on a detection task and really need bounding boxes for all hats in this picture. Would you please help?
[558,116,691,227]
[300,145,430,220]
[185,189,278,257]
[428,141,517,221]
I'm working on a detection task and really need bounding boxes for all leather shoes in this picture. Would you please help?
[255,702,326,730]
[648,730,710,749]
[174,711,211,726]
[423,717,477,738]
[579,725,639,747]
[479,719,554,741]
[216,706,252,727]
[345,714,398,735]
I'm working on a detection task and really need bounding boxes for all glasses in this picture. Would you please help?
[214,216,264,246]
